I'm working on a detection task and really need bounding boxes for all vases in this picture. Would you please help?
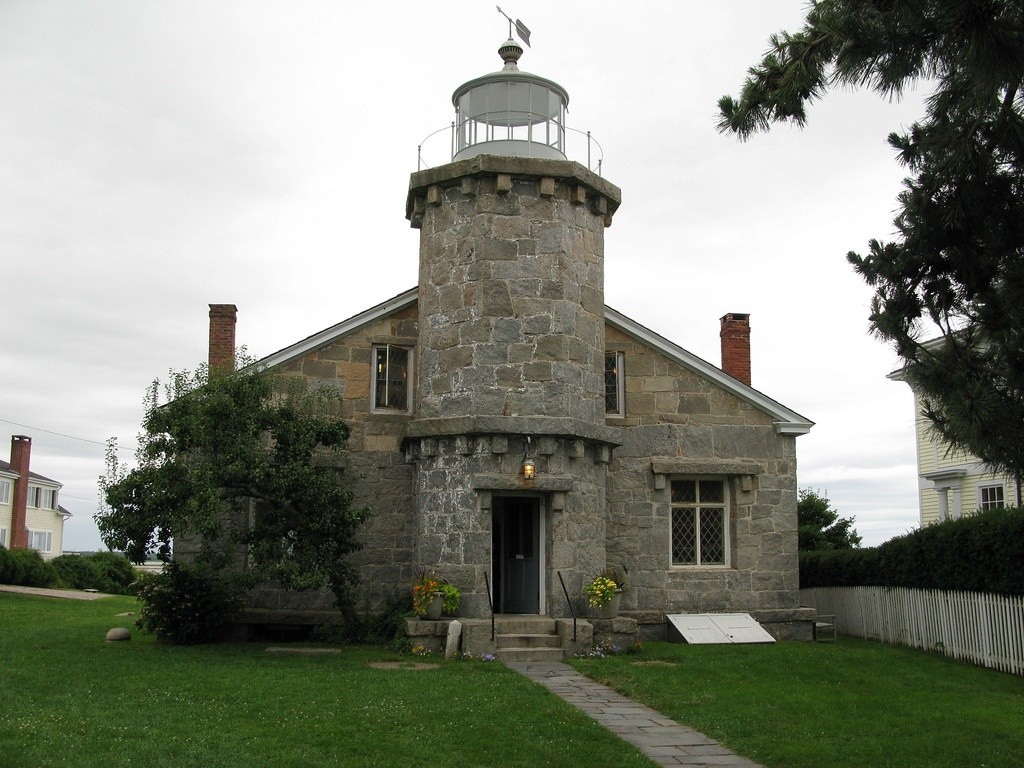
[416,591,446,620]
[583,588,622,618]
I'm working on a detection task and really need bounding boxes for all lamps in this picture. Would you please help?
[520,451,537,479]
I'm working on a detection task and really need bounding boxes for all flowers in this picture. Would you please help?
[583,562,629,611]
[409,564,463,619]
